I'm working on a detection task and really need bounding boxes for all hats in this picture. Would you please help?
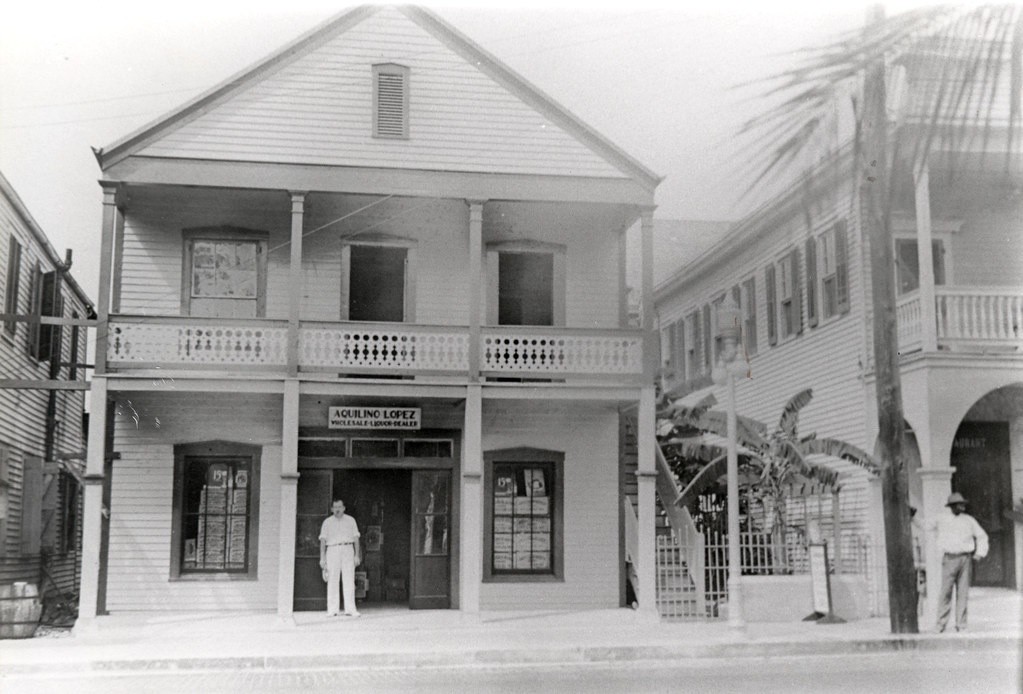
[943,491,969,507]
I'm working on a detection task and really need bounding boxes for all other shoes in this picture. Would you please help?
[327,610,340,617]
[344,608,359,617]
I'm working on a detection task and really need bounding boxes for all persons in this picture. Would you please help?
[910,492,989,633]
[318,498,361,617]
